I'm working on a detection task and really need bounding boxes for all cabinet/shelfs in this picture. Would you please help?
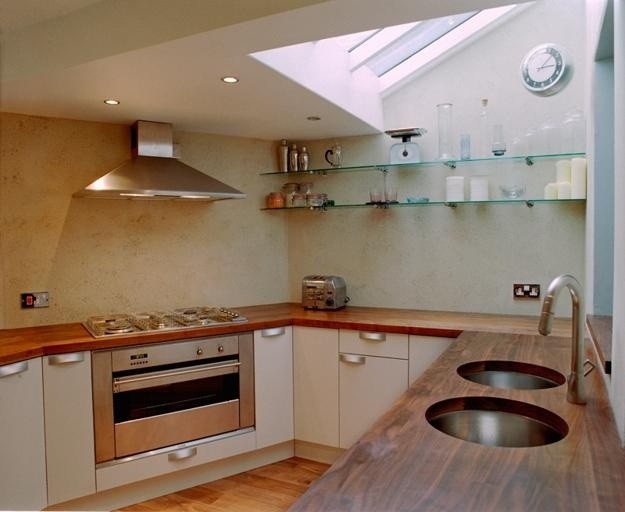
[0,357,44,510]
[338,329,458,468]
[261,153,585,210]
[43,324,294,511]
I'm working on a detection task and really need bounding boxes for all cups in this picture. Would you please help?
[492,124,506,155]
[370,183,397,203]
[544,157,587,199]
[324,144,344,168]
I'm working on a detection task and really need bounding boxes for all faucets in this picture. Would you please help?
[537,272,597,406]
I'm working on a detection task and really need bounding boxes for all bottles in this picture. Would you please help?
[266,182,328,208]
[278,139,308,174]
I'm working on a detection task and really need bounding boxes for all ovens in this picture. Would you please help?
[90,330,262,463]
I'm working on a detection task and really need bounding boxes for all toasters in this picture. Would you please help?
[301,275,349,311]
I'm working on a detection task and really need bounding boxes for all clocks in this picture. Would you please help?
[520,44,566,93]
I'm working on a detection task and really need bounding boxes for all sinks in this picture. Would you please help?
[423,396,570,448]
[457,359,567,390]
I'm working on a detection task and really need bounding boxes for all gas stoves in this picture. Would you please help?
[81,305,247,340]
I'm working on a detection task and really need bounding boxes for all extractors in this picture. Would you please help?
[71,120,247,204]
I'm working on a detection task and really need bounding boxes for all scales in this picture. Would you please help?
[384,128,426,164]
